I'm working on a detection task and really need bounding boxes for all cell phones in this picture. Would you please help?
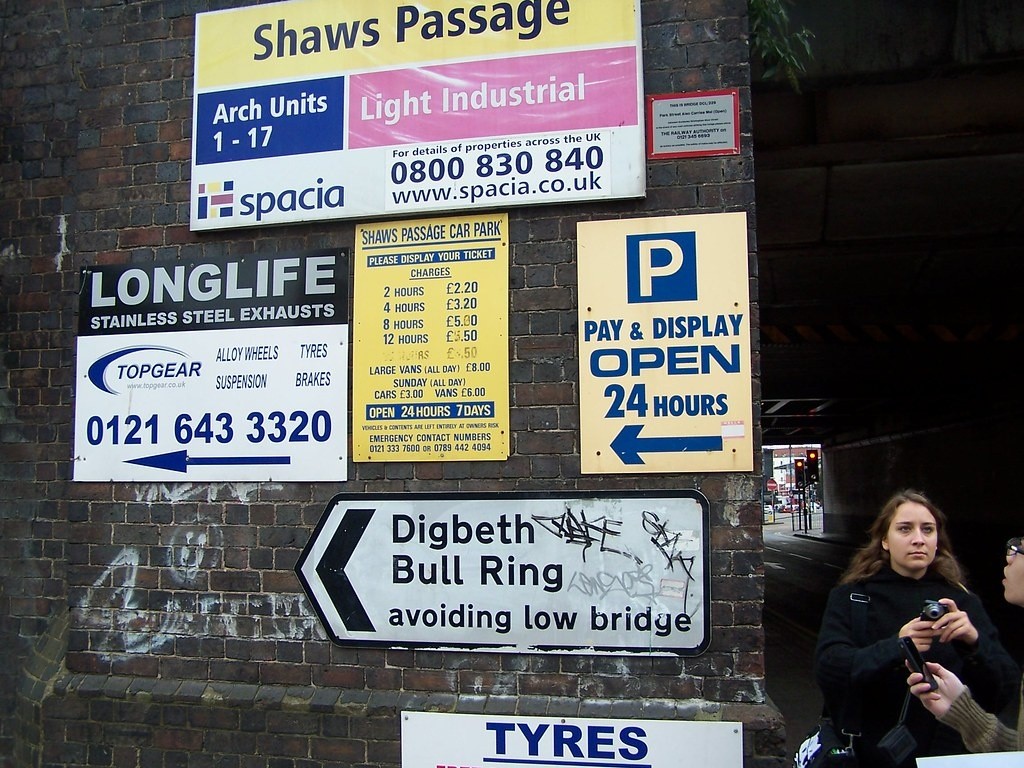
[899,636,939,692]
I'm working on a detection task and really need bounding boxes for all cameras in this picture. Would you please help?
[919,600,949,630]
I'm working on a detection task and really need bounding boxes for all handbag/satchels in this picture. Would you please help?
[792,723,863,768]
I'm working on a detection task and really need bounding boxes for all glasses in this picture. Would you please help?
[1006,536,1023,561]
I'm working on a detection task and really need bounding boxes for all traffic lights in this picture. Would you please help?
[795,459,805,488]
[807,450,819,481]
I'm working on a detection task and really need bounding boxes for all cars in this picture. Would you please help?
[778,505,799,513]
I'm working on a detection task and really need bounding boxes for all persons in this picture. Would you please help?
[814,488,1020,768]
[903,536,1024,755]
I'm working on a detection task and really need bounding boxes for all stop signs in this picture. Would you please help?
[767,479,777,491]
